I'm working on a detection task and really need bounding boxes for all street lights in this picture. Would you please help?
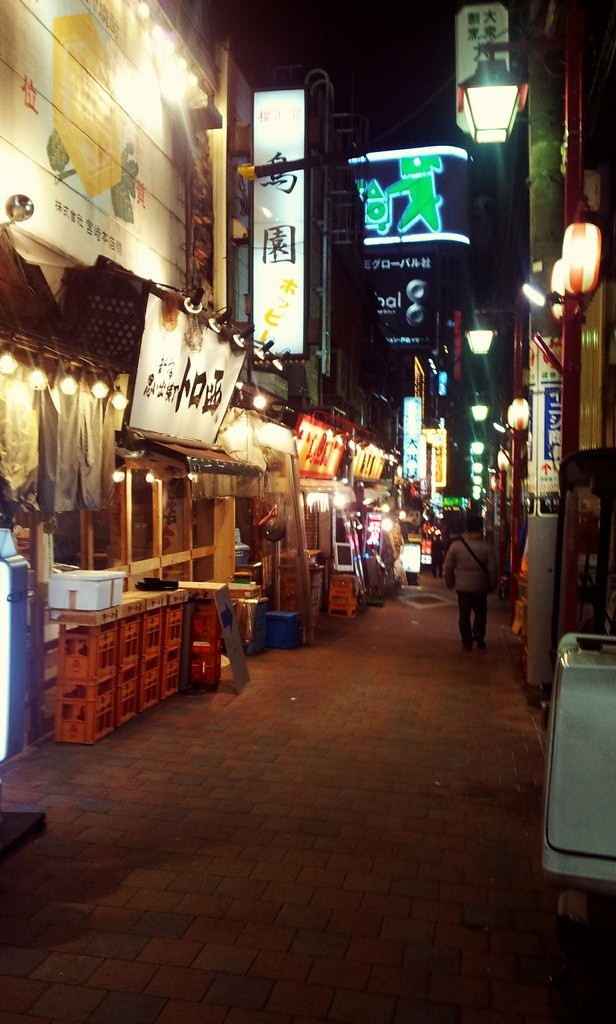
[545,189,604,468]
[507,394,529,627]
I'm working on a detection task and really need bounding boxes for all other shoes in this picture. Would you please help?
[475,638,486,649]
[461,639,473,650]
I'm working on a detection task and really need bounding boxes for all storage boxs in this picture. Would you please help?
[117,662,138,728]
[54,679,115,744]
[193,600,222,639]
[122,591,167,610]
[167,589,188,605]
[56,624,117,680]
[139,650,161,714]
[119,614,139,668]
[164,603,183,646]
[284,570,360,620]
[48,608,117,627]
[179,581,226,600]
[266,611,304,648]
[48,570,127,609]
[191,636,221,686]
[241,602,267,654]
[141,607,162,657]
[227,582,261,598]
[118,596,146,618]
[159,645,178,700]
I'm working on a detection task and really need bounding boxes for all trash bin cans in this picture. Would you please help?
[539,633,616,882]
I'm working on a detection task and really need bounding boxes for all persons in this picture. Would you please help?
[443,516,499,652]
[430,534,444,578]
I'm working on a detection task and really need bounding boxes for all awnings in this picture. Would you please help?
[124,427,264,501]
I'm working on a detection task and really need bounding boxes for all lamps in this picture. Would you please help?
[561,220,603,295]
[254,336,275,360]
[492,420,511,433]
[208,306,233,333]
[271,401,287,410]
[522,281,565,306]
[358,442,371,450]
[27,344,49,390]
[156,281,205,314]
[91,363,110,399]
[273,347,292,370]
[0,335,17,374]
[233,323,256,348]
[59,358,79,396]
[328,426,349,439]
[112,370,129,410]
[349,439,364,451]
[337,431,360,445]
